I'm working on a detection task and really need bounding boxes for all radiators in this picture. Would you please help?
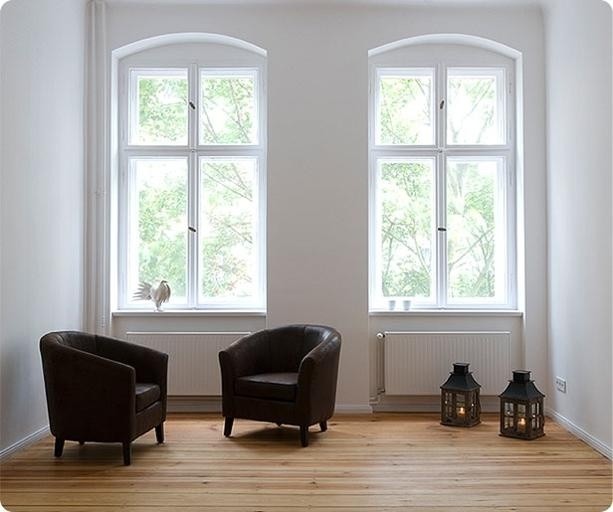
[376,331,512,396]
[119,329,252,400]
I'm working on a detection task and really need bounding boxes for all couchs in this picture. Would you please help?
[217,322,342,447]
[38,328,169,467]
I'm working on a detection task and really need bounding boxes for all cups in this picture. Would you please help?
[387,301,396,310]
[402,300,411,310]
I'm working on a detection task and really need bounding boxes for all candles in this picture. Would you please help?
[517,417,525,433]
[456,407,466,422]
